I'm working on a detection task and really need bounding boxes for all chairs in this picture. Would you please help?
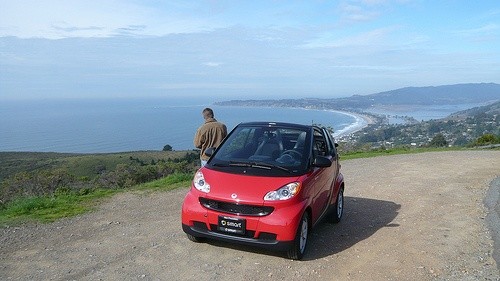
[253,130,284,158]
[293,131,307,156]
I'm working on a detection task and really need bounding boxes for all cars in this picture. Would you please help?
[181,122,345,260]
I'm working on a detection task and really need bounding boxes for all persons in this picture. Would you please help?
[195,108,228,168]
